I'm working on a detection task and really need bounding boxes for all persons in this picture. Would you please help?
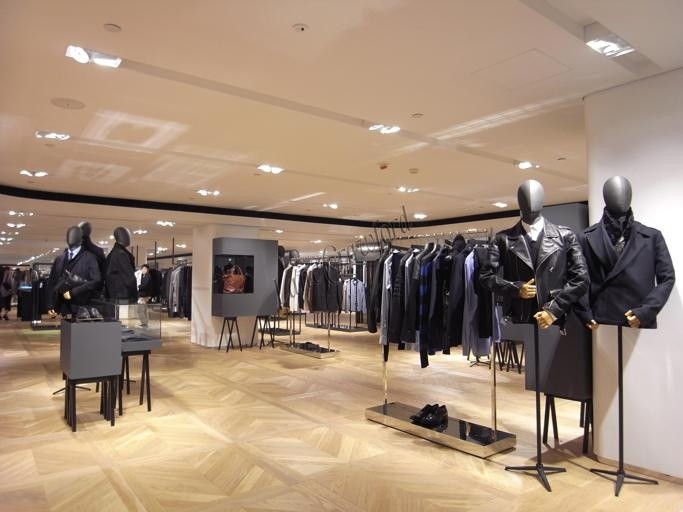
[101,226,139,306]
[572,174,676,332]
[277,243,287,294]
[45,224,103,319]
[134,264,154,328]
[0,264,14,321]
[11,267,21,303]
[63,219,105,270]
[475,178,590,331]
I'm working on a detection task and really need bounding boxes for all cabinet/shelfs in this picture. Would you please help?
[213,236,279,316]
[92,297,162,416]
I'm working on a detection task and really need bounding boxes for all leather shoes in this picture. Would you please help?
[410,404,448,426]
[0,313,10,320]
[78,306,102,319]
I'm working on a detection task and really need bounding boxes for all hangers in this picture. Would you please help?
[380,227,488,260]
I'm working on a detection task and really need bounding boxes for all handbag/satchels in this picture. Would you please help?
[4,273,12,291]
[222,263,247,294]
[51,267,93,306]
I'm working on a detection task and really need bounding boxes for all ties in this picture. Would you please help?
[69,250,74,261]
